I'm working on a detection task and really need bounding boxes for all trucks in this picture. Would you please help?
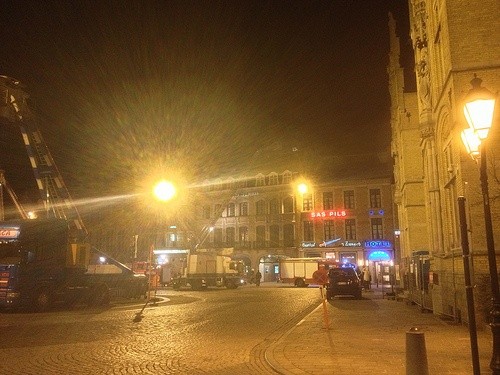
[0,218,110,313]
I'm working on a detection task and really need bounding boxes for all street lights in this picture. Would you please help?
[457,73,500,375]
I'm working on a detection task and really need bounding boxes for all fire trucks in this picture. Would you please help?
[278,257,339,287]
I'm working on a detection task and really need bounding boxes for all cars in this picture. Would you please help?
[325,266,363,300]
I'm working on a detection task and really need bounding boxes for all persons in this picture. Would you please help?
[255,270,262,286]
[249,268,255,285]
[362,266,371,292]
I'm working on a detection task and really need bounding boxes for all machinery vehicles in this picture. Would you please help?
[178,144,288,291]
